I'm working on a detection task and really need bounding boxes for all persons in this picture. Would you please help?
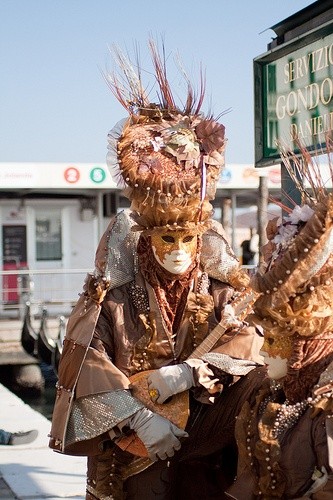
[48,38,333,500]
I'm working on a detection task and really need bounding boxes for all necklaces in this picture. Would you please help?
[246,383,296,496]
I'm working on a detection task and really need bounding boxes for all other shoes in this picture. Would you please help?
[8,430,37,444]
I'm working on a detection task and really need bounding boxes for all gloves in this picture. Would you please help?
[146,363,201,404]
[126,408,189,461]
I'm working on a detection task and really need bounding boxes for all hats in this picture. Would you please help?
[106,117,229,231]
[243,193,333,336]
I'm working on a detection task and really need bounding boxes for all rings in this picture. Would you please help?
[149,387,160,399]
